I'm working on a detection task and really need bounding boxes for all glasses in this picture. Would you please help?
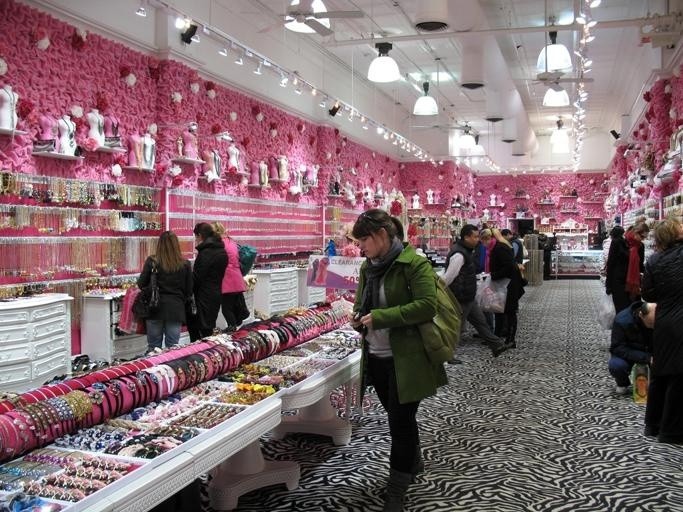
[638,232,647,239]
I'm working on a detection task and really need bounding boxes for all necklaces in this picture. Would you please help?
[177,188,319,254]
[2,87,15,130]
[0,171,162,280]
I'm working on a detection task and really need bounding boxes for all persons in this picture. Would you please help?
[1,85,20,130]
[182,126,247,180]
[128,130,157,170]
[39,111,81,156]
[250,156,318,188]
[352,209,449,512]
[86,108,124,148]
[361,185,443,211]
[441,224,529,365]
[134,221,249,353]
[606,217,683,446]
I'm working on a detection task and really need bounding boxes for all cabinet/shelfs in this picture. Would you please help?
[405,211,451,257]
[604,193,682,265]
[0,171,161,298]
[552,249,601,280]
[324,206,364,257]
[166,188,323,271]
[553,219,589,251]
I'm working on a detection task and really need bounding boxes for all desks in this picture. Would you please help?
[80,275,257,368]
[0,300,365,511]
[252,266,326,320]
[0,291,75,396]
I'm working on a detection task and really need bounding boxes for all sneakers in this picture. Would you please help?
[415,445,425,472]
[616,385,628,394]
[382,478,402,512]
[643,423,660,436]
[493,344,514,358]
[447,359,462,364]
[656,430,683,443]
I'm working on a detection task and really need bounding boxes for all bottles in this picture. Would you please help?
[632,361,649,403]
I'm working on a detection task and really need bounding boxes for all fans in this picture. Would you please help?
[509,0,594,93]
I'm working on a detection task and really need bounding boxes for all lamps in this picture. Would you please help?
[536,17,571,155]
[572,0,602,160]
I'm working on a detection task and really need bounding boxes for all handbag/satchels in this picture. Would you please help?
[185,293,197,321]
[404,261,462,365]
[132,254,157,322]
[228,237,257,276]
